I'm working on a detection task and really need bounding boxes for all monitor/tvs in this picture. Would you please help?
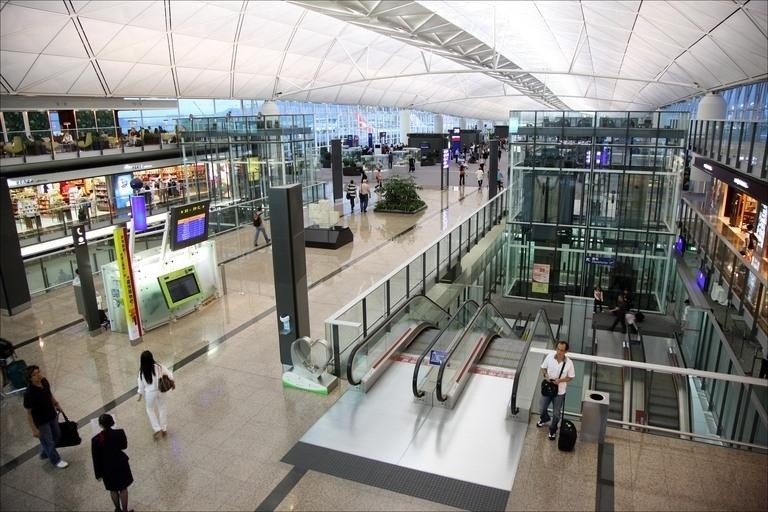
[170,199,209,251]
[157,265,203,309]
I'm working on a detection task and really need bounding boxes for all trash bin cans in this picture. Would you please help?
[579,390,610,442]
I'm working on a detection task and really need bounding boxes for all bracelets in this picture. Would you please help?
[55,401,58,406]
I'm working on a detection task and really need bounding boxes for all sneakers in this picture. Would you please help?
[548,431,556,440]
[56,460,69,469]
[536,420,546,427]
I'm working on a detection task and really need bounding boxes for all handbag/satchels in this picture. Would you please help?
[55,411,80,447]
[541,379,559,397]
[157,366,172,393]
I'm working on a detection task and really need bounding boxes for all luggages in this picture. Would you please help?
[558,393,576,452]
[6,360,27,389]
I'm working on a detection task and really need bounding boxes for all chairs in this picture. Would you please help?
[0,123,179,162]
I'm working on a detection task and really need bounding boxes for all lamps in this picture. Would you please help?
[696,88,732,122]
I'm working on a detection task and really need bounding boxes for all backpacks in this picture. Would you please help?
[253,212,262,227]
[0,338,17,362]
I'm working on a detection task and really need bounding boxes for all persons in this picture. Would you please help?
[137,351,175,439]
[409,157,415,172]
[346,142,404,214]
[60,179,96,219]
[683,299,690,328]
[138,171,183,204]
[746,224,757,264]
[254,207,270,246]
[449,140,509,191]
[537,341,575,439]
[52,125,186,151]
[91,414,133,512]
[738,251,749,288]
[24,365,69,469]
[593,285,644,349]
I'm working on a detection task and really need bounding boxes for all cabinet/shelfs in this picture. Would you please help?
[6,164,210,224]
